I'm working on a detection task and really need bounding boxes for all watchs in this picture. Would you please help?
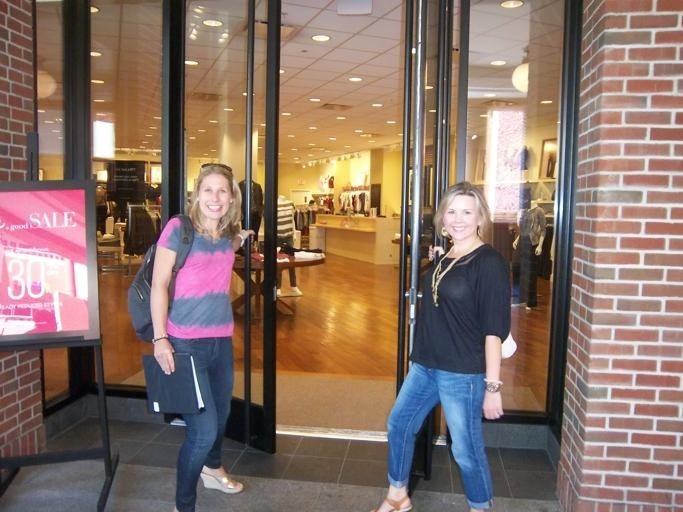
[485,379,503,393]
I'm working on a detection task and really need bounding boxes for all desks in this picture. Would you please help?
[231,250,324,320]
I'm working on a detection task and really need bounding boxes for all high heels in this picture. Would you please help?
[201,466,244,494]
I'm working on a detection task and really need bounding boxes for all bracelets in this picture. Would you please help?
[152,335,168,343]
[237,234,244,247]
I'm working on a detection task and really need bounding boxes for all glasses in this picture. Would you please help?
[201,164,232,172]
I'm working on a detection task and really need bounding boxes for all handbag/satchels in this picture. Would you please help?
[127,244,156,343]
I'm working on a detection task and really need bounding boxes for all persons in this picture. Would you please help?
[513,200,546,310]
[276,195,300,296]
[150,163,256,512]
[238,180,263,242]
[370,181,511,512]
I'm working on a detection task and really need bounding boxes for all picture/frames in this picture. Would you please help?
[538,138,557,179]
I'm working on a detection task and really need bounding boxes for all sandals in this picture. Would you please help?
[371,495,413,512]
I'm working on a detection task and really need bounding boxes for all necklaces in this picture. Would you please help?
[432,236,480,308]
